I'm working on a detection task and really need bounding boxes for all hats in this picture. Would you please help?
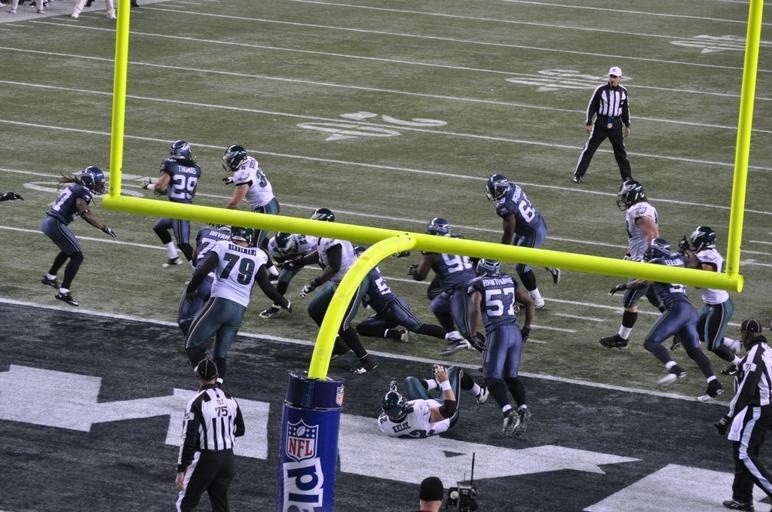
[197,359,218,380]
[741,319,762,333]
[609,66,622,76]
[420,477,443,501]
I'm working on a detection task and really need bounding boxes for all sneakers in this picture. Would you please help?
[475,386,489,405]
[502,408,531,438]
[697,366,739,402]
[724,501,754,511]
[351,358,379,376]
[443,339,468,356]
[259,305,282,318]
[658,368,686,385]
[162,257,182,267]
[41,276,78,306]
[552,268,559,283]
[399,330,410,343]
[600,334,628,348]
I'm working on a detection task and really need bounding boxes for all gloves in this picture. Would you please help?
[103,226,117,238]
[300,284,316,295]
[470,336,486,351]
[521,325,529,342]
[719,415,730,434]
[186,290,197,305]
[222,177,234,184]
[610,283,627,295]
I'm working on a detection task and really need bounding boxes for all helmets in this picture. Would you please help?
[644,226,716,261]
[223,145,246,171]
[312,208,334,222]
[231,226,293,254]
[486,174,509,201]
[170,140,191,160]
[617,180,646,211]
[382,391,405,413]
[428,217,449,235]
[81,165,104,195]
[476,258,502,277]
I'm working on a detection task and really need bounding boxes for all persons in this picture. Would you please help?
[608,239,725,403]
[220,143,280,248]
[684,225,746,376]
[714,318,772,512]
[0,191,25,202]
[143,140,201,270]
[40,166,116,306]
[174,359,245,512]
[571,65,632,186]
[598,179,684,353]
[484,172,561,310]
[419,476,444,512]
[0,0,140,20]
